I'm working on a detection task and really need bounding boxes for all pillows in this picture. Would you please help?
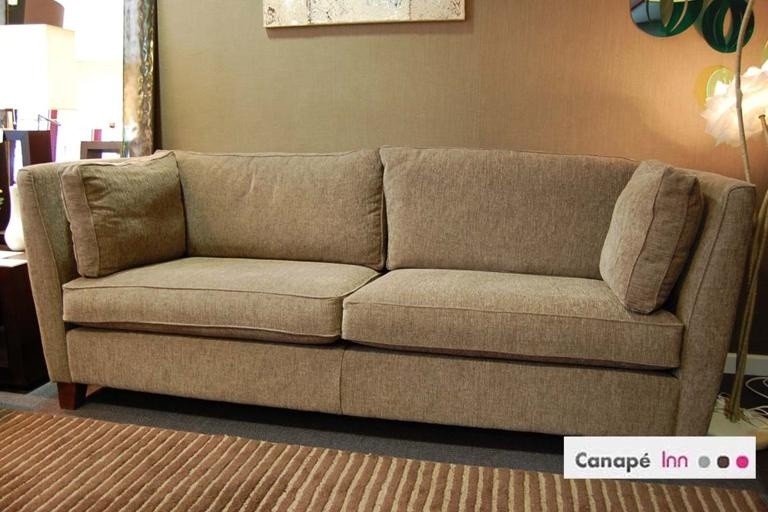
[58,147,703,314]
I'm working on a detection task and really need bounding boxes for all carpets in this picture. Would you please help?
[0,405,767,512]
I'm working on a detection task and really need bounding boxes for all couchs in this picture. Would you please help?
[16,144,756,436]
